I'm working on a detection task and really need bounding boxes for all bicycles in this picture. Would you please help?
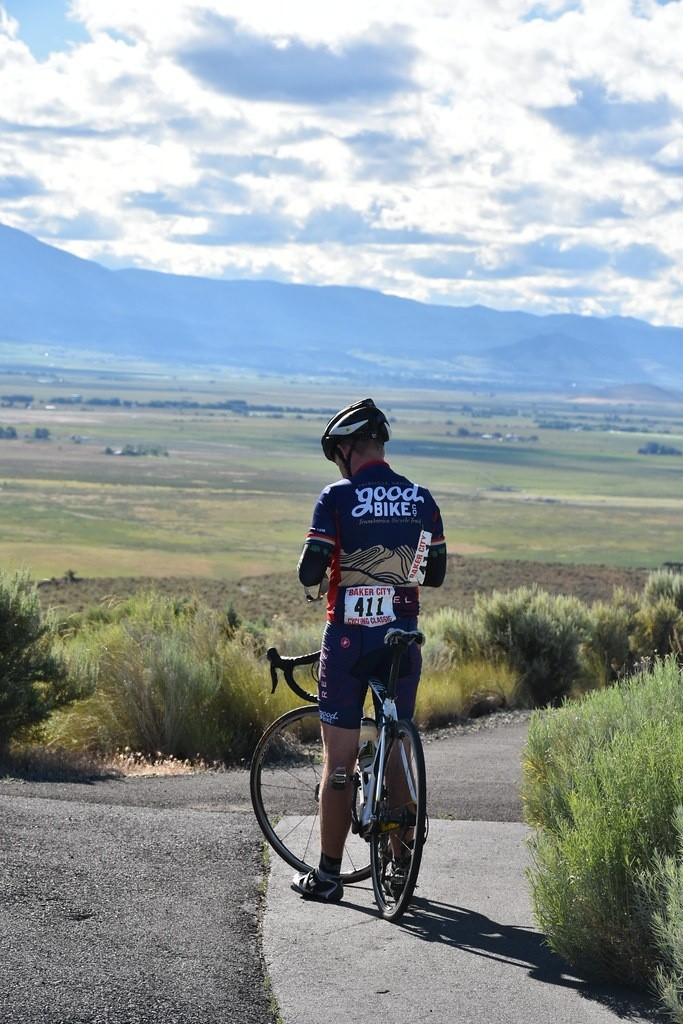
[250,628,432,920]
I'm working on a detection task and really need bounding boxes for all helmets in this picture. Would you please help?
[321,398,393,462]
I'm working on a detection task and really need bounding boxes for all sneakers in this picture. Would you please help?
[290,867,344,902]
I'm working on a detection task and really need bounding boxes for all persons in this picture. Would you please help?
[289,397,447,902]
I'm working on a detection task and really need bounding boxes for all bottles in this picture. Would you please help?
[360,740,376,798]
